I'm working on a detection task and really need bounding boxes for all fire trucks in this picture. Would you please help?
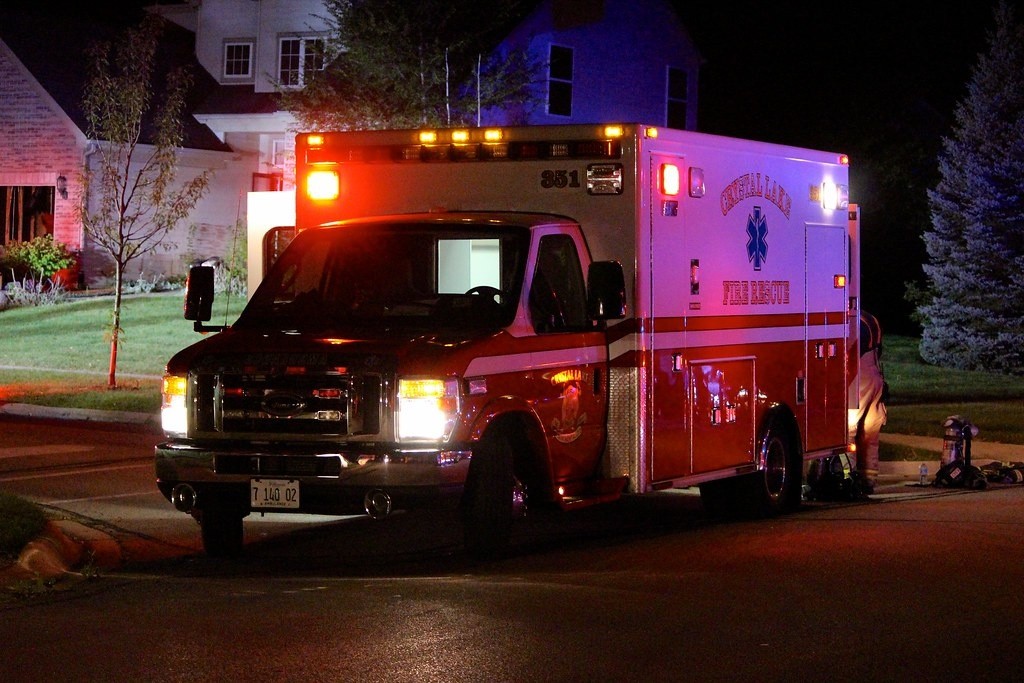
[153,122,860,564]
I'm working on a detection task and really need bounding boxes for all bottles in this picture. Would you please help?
[919,463,928,485]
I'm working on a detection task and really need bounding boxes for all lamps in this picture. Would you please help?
[57,176,66,195]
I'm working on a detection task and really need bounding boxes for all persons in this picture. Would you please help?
[842,310,888,486]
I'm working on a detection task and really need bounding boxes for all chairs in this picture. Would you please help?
[500,248,563,333]
